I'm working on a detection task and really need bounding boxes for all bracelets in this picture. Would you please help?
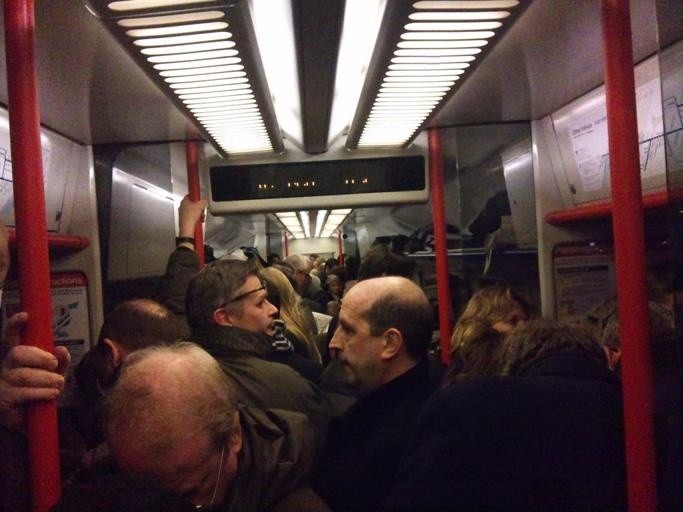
[172,235,197,245]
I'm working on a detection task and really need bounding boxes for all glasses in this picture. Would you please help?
[299,271,311,282]
[218,280,266,308]
[180,445,228,512]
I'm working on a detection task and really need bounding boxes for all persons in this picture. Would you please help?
[281,242,405,314]
[380,318,629,512]
[0,216,29,511]
[162,192,317,382]
[203,239,281,268]
[601,302,682,512]
[0,310,324,510]
[308,274,442,512]
[255,265,322,371]
[184,259,337,452]
[94,295,184,391]
[433,282,537,386]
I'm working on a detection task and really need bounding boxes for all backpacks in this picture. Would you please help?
[468,190,511,246]
[407,220,458,254]
